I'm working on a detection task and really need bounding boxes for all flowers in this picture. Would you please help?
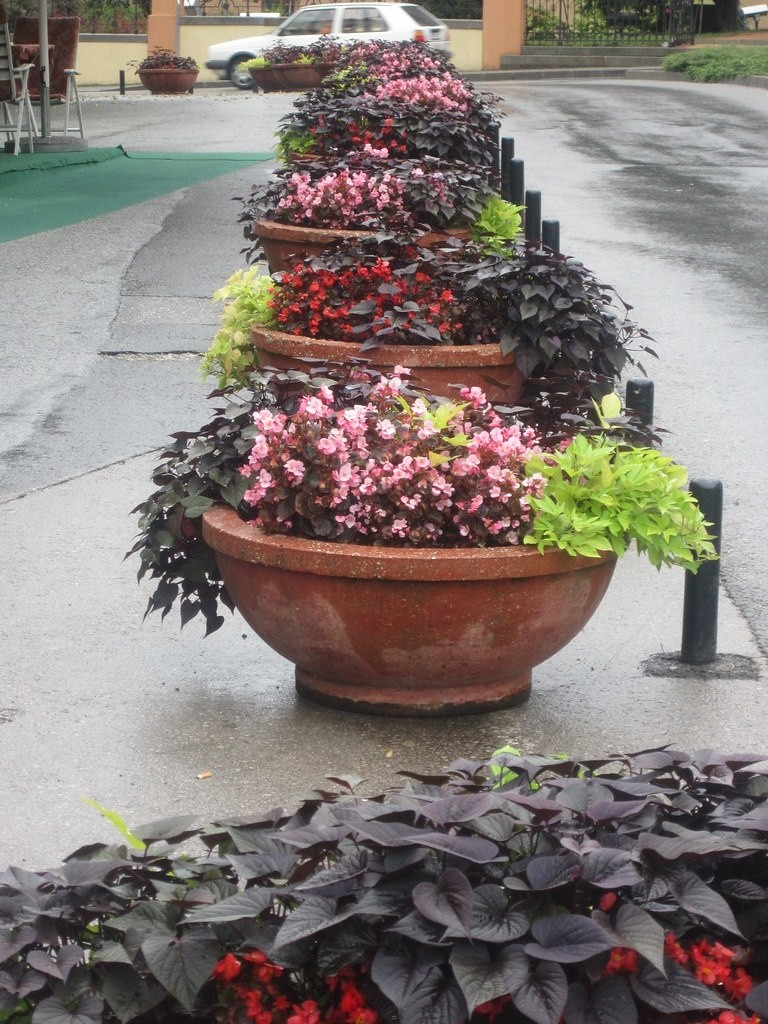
[196,192,658,420]
[231,33,530,267]
[120,362,719,639]
[125,45,199,74]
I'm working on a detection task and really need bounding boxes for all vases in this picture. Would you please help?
[250,217,469,274]
[199,504,624,716]
[269,63,324,90]
[248,67,286,93]
[138,67,199,96]
[315,61,338,75]
[251,319,524,405]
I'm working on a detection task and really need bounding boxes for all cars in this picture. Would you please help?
[204,1,455,90]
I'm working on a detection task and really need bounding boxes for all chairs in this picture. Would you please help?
[3,16,84,141]
[0,5,35,157]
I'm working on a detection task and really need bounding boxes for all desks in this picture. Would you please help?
[6,43,54,140]
